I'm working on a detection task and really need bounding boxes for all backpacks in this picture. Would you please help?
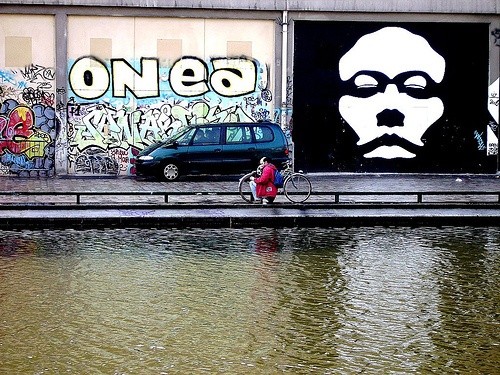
[266,166,284,187]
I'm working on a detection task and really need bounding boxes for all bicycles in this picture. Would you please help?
[237,160,311,203]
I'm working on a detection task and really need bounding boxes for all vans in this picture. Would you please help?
[136,122,290,179]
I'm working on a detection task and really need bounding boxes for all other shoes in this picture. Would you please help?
[252,200,262,204]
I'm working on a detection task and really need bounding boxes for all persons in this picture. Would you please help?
[204,130,216,142]
[249,156,278,204]
[194,129,210,142]
